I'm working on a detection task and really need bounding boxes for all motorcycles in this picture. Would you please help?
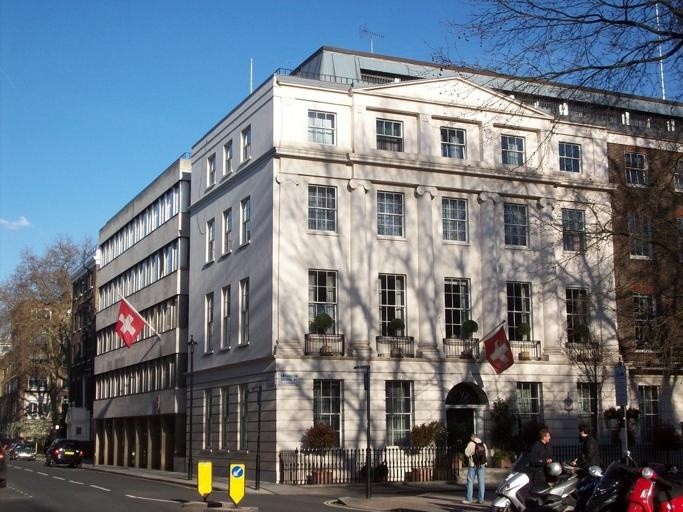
[492,449,683,512]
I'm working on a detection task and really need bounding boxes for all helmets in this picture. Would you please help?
[563,461,581,473]
[547,461,563,477]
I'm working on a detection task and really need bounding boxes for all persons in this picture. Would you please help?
[567,422,601,511]
[527,428,553,473]
[461,433,488,505]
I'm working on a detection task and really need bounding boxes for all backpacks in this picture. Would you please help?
[473,442,486,465]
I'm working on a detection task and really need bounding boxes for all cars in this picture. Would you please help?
[46,438,84,468]
[0,440,36,488]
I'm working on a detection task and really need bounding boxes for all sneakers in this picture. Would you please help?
[461,500,471,504]
[472,499,484,504]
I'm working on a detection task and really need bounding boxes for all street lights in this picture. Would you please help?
[353,365,372,499]
[185,335,198,479]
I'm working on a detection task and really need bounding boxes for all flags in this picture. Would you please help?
[114,298,145,348]
[483,326,513,374]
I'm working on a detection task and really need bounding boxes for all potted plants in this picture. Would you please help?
[403,422,451,483]
[604,406,641,429]
[300,422,335,484]
[313,311,591,363]
[491,397,517,468]
